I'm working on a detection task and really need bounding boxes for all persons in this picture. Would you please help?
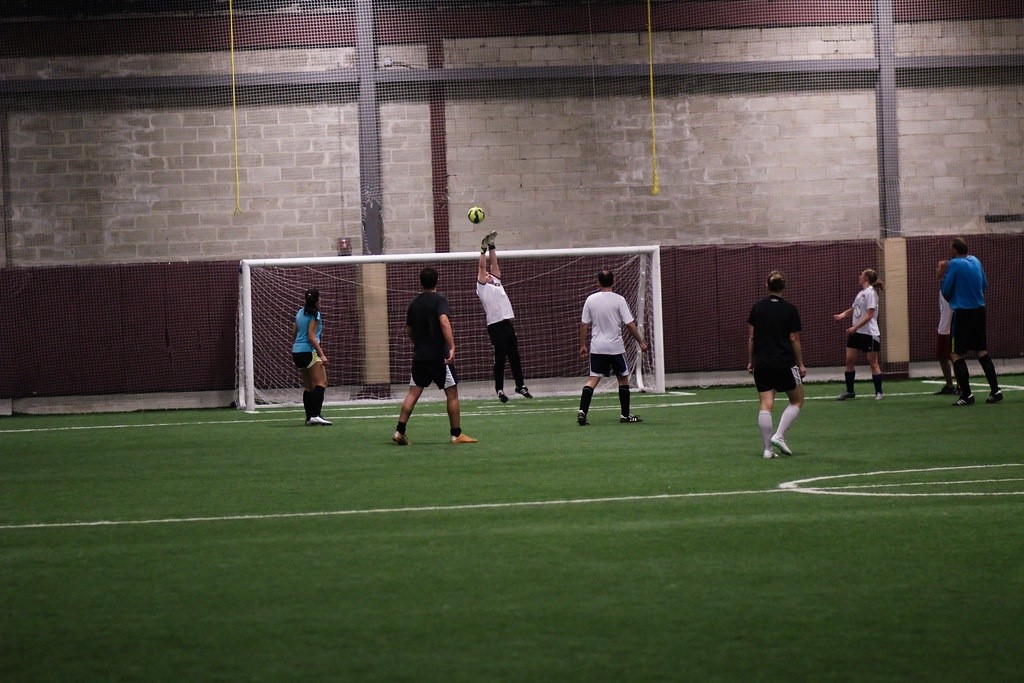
[834,269,884,401]
[475,231,532,403]
[940,237,1002,406]
[392,268,478,446]
[934,261,961,396]
[291,289,333,425]
[747,270,807,459]
[576,271,648,426]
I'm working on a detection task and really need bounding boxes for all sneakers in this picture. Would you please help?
[498,392,508,403]
[392,431,408,445]
[620,414,643,422]
[952,393,975,406]
[450,433,477,443]
[953,385,963,395]
[770,434,792,455]
[763,449,778,459]
[515,386,533,399]
[935,386,955,396]
[309,415,332,425]
[578,409,589,425]
[305,420,311,426]
[986,387,1003,402]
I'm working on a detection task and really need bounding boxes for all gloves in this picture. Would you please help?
[486,230,497,250]
[481,238,488,254]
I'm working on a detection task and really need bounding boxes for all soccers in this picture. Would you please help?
[467,207,486,224]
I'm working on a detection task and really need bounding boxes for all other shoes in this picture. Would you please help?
[839,392,855,400]
[875,391,883,400]
